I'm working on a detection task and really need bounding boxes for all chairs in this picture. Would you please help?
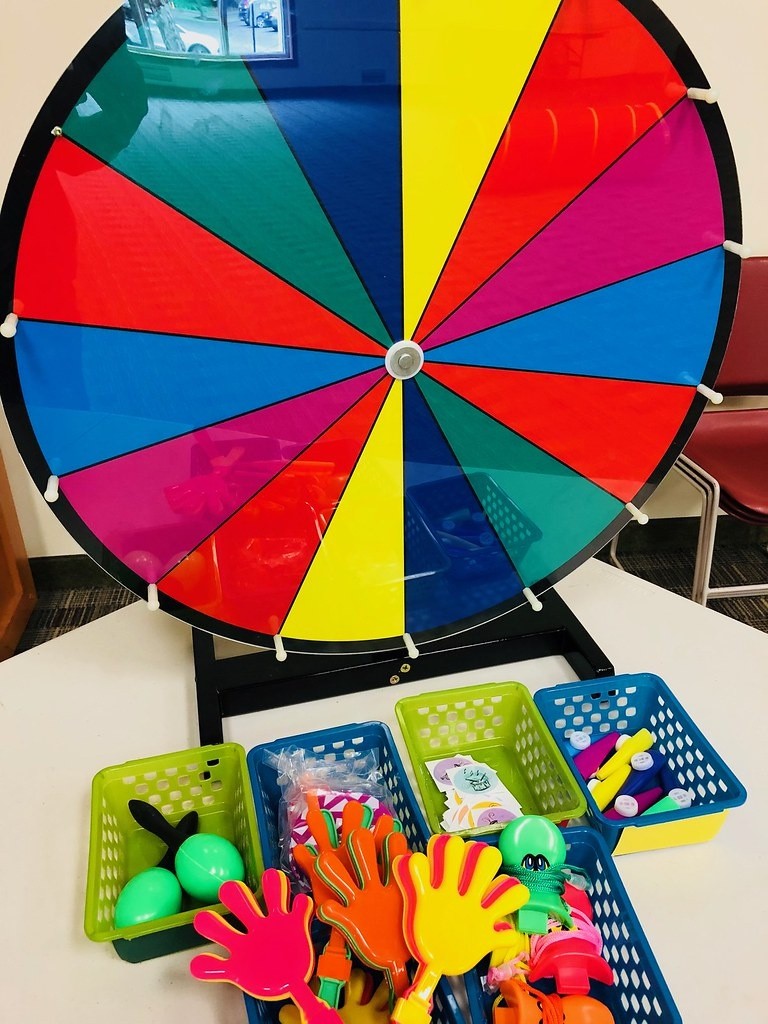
[611,256,768,608]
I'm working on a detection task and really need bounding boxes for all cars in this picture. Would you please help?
[237,0,279,32]
[124,6,221,55]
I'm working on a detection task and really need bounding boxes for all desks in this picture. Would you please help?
[0,557,768,1024]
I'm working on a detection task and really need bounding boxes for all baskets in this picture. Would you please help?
[81,671,747,1024]
